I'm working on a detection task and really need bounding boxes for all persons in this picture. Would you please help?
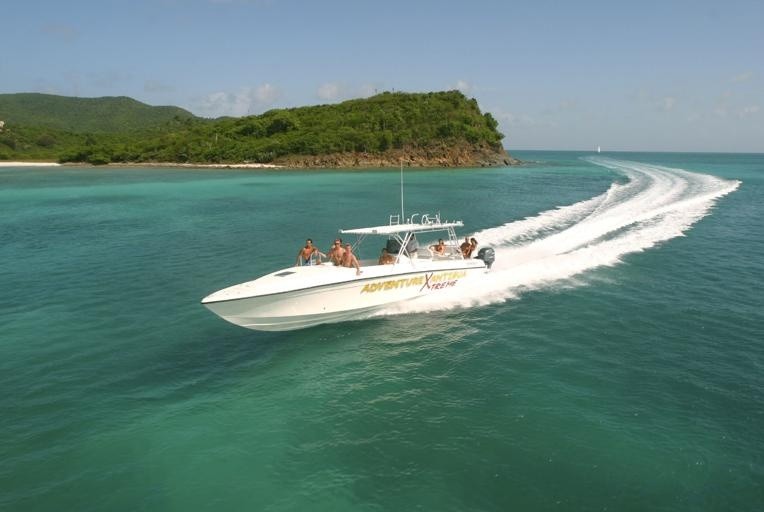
[295,237,361,276]
[379,248,398,265]
[431,239,445,255]
[403,232,418,259]
[461,237,479,259]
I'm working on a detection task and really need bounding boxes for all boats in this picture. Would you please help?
[200,159,496,334]
